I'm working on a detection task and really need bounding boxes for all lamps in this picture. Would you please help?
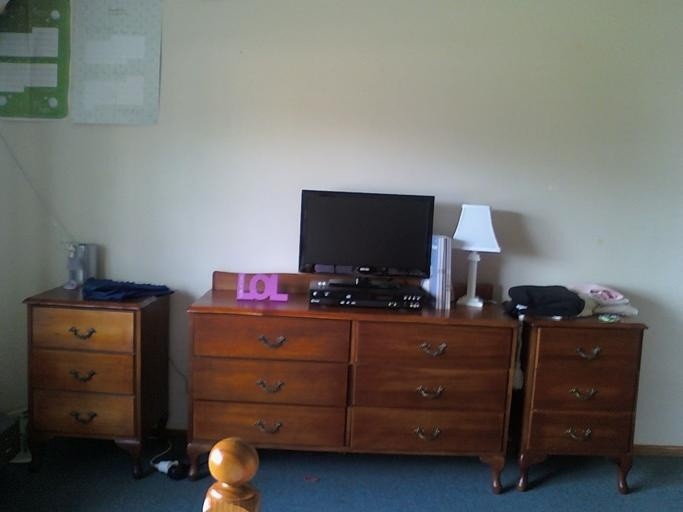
[451,204,501,309]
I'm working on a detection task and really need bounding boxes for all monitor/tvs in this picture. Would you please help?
[299,189,435,287]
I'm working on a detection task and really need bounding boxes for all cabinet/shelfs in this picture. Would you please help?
[24,284,170,480]
[187,272,523,492]
[503,301,646,493]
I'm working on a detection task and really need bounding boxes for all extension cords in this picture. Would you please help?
[155,461,178,474]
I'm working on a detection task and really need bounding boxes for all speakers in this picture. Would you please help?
[67,243,98,287]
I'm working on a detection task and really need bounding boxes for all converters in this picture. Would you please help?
[168,464,190,480]
